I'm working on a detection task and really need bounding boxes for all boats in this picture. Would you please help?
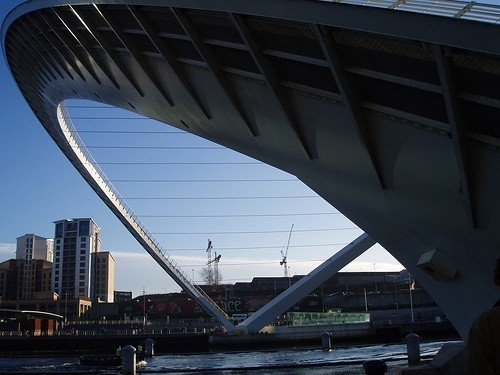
[80,347,148,369]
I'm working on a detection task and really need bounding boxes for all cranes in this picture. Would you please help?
[205,224,295,284]
